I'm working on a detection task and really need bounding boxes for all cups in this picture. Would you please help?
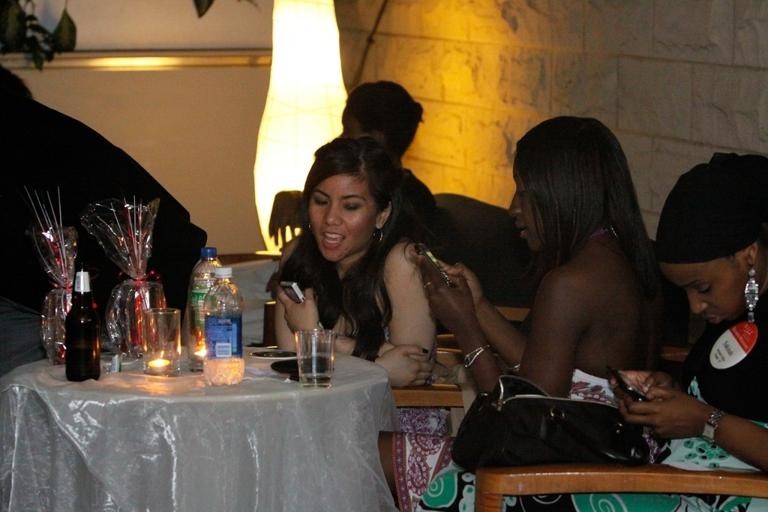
[297,330,336,389]
[141,306,180,374]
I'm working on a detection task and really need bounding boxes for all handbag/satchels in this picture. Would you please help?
[450,374,648,475]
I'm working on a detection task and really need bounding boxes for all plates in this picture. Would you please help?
[248,351,297,360]
[271,357,298,381]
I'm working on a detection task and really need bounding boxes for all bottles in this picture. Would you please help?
[42,263,166,382]
[191,247,244,388]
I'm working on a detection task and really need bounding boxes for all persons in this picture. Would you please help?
[341,83,438,240]
[271,134,434,386]
[416,151,766,510]
[380,117,655,510]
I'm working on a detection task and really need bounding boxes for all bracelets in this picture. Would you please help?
[465,346,486,364]
[350,346,376,360]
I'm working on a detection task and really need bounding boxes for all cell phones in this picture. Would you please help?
[280,281,324,329]
[414,243,456,288]
[606,365,652,402]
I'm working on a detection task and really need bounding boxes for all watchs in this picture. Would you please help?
[703,410,722,442]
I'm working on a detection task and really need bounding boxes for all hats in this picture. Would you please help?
[655,153,767,262]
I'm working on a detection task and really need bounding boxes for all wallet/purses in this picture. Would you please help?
[271,358,327,373]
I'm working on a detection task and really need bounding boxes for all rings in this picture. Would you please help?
[423,280,432,292]
